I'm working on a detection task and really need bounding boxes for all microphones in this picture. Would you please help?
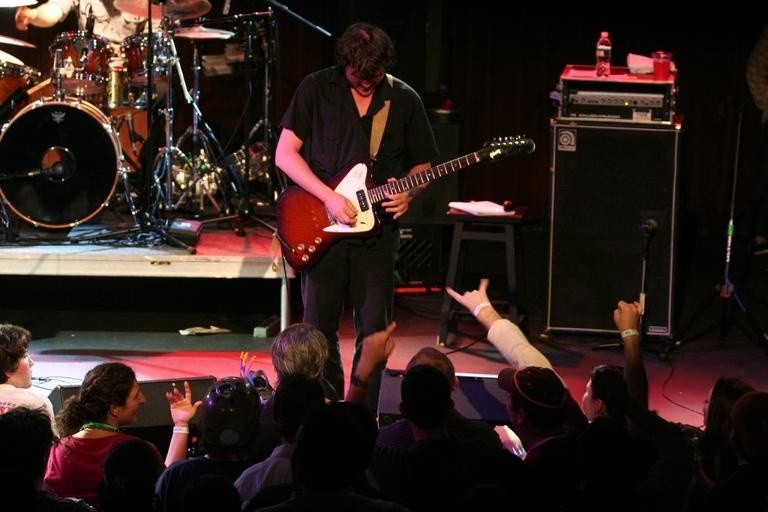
[638,219,659,238]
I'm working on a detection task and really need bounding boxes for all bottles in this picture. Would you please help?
[596,31,611,78]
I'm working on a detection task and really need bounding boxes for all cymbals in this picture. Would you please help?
[114,1,211,22]
[173,26,235,40]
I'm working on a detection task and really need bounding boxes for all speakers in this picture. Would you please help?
[551,123,667,333]
[47,376,217,460]
[376,368,509,442]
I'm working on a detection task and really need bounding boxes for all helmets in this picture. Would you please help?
[200,377,259,446]
[731,392,768,432]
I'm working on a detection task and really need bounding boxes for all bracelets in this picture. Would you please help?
[171,426,191,434]
[620,329,639,339]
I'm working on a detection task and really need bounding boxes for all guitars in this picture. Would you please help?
[279,136,536,272]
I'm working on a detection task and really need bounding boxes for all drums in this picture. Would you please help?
[122,32,173,78]
[0,96,122,228]
[0,50,25,105]
[51,31,110,82]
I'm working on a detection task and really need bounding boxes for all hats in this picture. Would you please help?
[497,366,564,412]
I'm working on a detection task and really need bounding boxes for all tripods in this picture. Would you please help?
[174,40,229,217]
[152,15,232,232]
[202,12,275,234]
[241,0,331,205]
[70,0,197,255]
[664,107,764,356]
[591,238,670,361]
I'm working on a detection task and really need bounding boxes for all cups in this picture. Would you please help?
[652,52,671,80]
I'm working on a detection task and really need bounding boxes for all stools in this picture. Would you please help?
[437,212,522,349]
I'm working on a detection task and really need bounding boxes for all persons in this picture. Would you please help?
[346,322,461,470]
[612,301,756,490]
[0,407,98,511]
[256,434,404,511]
[0,322,61,441]
[16,1,160,78]
[381,367,483,512]
[275,22,437,401]
[41,362,203,506]
[446,277,591,499]
[583,364,626,424]
[721,391,768,512]
[103,436,159,512]
[263,322,330,429]
[154,378,267,511]
